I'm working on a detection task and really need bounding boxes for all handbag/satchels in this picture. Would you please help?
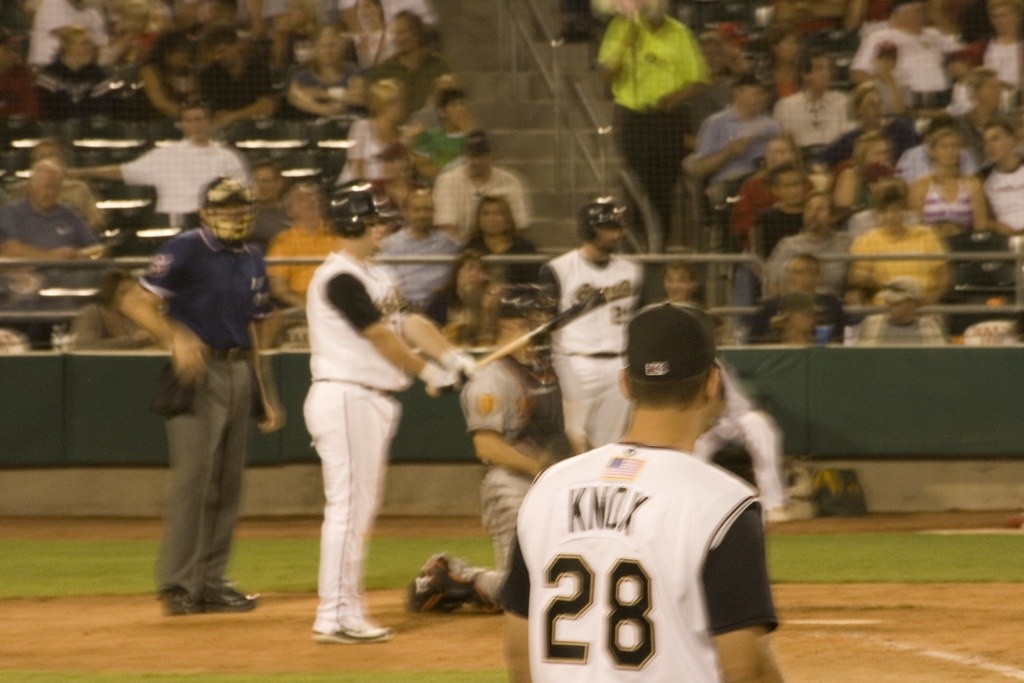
[146,363,194,419]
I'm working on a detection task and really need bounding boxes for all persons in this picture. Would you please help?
[495,302,781,683]
[0,0,1024,644]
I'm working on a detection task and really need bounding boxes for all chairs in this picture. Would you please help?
[1,116,1012,346]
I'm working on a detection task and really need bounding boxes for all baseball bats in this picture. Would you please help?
[447,279,635,385]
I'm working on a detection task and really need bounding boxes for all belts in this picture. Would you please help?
[215,349,245,365]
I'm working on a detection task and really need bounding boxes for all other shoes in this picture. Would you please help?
[405,553,452,611]
[312,622,392,645]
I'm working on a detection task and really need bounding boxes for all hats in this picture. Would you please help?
[876,275,921,303]
[626,302,715,383]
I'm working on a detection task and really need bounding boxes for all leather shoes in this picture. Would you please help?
[160,584,258,615]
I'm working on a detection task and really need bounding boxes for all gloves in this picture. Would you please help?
[419,363,462,398]
[443,349,477,377]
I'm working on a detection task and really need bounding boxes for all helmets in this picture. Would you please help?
[327,179,394,239]
[578,197,626,241]
[201,176,253,244]
[498,284,556,322]
